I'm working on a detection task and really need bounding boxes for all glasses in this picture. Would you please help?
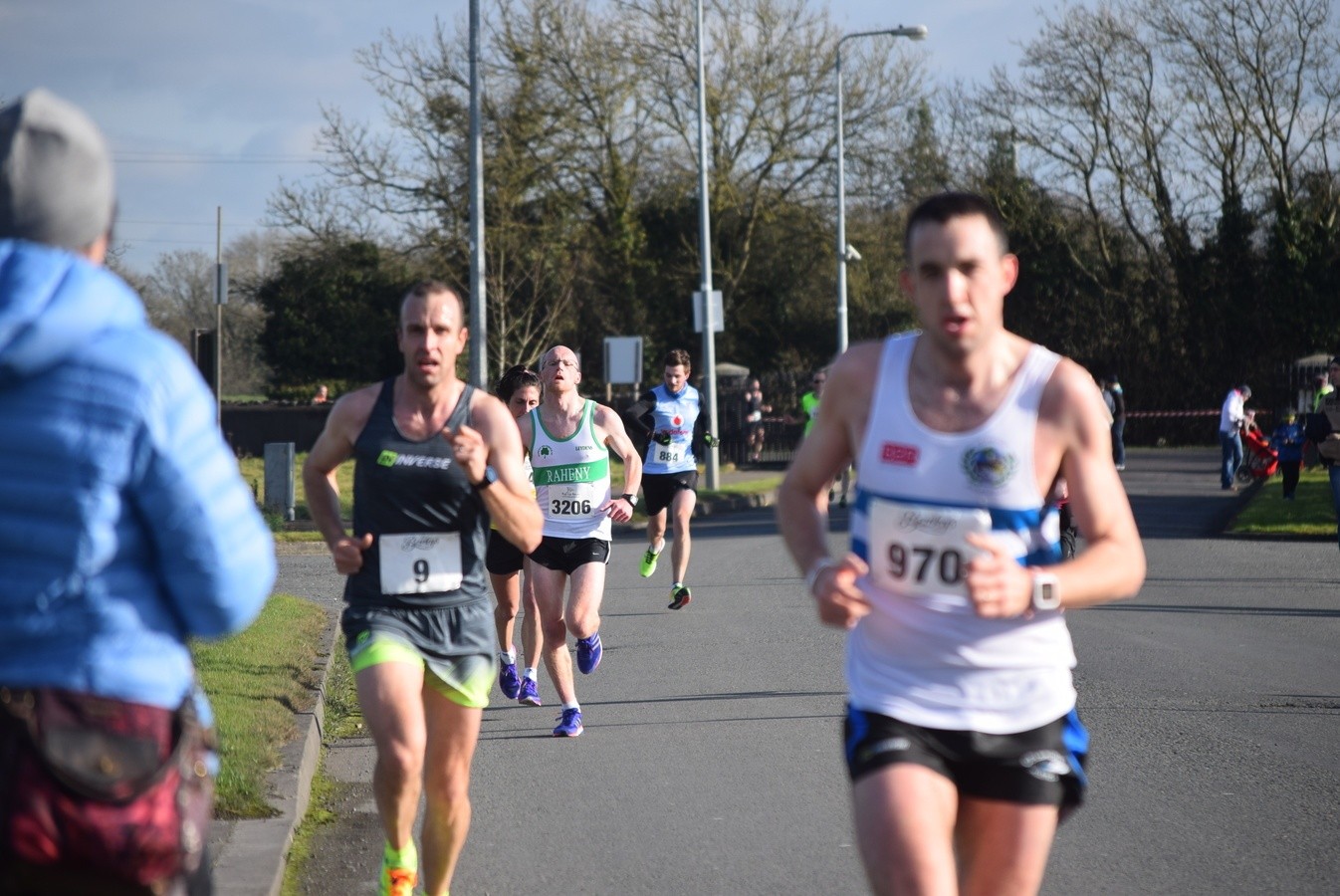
[813,379,826,384]
[541,358,579,372]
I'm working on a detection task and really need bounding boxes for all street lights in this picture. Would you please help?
[833,22,930,357]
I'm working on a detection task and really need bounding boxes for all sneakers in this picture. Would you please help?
[499,644,521,700]
[639,537,665,578]
[551,708,584,737]
[380,842,418,896]
[518,675,541,707]
[575,631,602,675]
[667,586,691,610]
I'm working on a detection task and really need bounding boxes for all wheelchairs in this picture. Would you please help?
[1057,505,1081,562]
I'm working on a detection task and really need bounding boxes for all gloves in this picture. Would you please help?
[701,432,712,448]
[653,431,671,446]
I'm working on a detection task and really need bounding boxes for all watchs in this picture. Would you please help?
[1024,561,1064,619]
[470,465,498,490]
[622,494,639,507]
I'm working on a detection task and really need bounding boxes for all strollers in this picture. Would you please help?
[1236,425,1280,484]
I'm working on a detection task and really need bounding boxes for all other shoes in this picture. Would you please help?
[1115,460,1338,502]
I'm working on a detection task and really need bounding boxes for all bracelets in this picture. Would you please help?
[804,555,840,596]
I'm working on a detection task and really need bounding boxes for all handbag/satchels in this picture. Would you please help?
[2,678,222,896]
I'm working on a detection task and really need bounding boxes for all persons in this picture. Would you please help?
[1313,364,1338,415]
[726,360,861,520]
[772,193,1152,894]
[1110,376,1129,471]
[473,365,547,708]
[621,350,713,612]
[502,345,643,737]
[309,385,329,409]
[302,273,546,895]
[1094,377,1117,420]
[1273,409,1307,499]
[0,87,287,894]
[1312,357,1340,547]
[1218,385,1251,491]
